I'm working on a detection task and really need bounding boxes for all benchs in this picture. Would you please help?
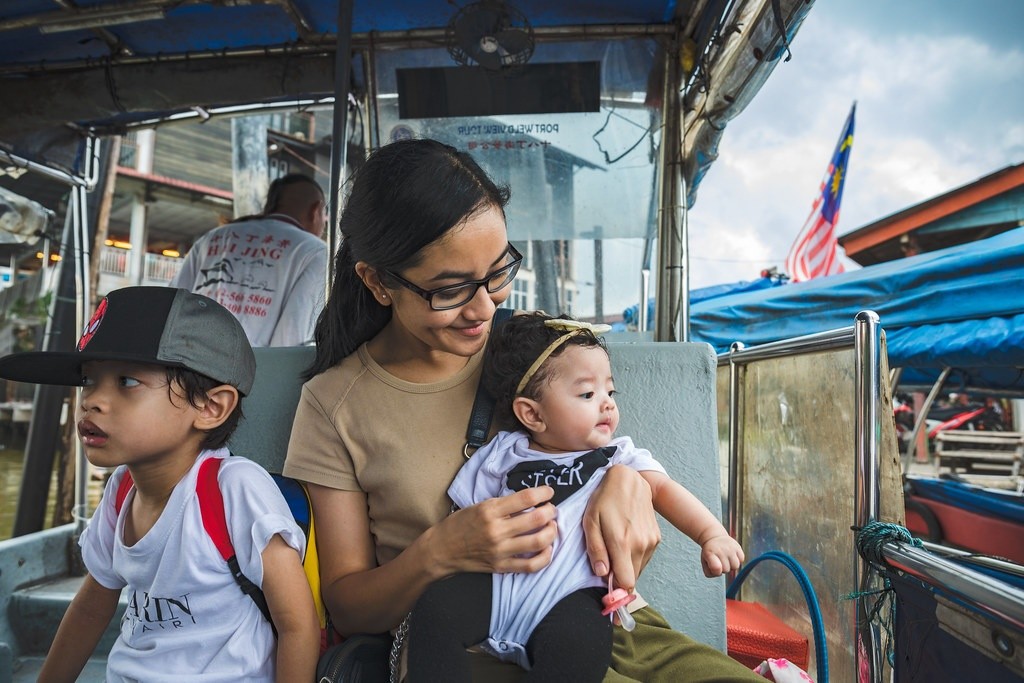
[216,343,728,654]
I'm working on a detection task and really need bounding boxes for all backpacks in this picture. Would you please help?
[116,458,327,653]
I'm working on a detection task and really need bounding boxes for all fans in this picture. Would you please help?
[444,0,537,80]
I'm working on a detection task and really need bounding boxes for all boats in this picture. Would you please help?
[834,157,1024,677]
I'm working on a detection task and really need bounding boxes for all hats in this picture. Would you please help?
[1,285,257,397]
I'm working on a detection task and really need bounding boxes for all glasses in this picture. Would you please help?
[375,241,524,311]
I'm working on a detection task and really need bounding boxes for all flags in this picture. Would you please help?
[784,101,858,282]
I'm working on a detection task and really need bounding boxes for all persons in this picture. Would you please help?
[401,309,746,683]
[170,171,332,349]
[278,136,778,683]
[0,287,326,683]
